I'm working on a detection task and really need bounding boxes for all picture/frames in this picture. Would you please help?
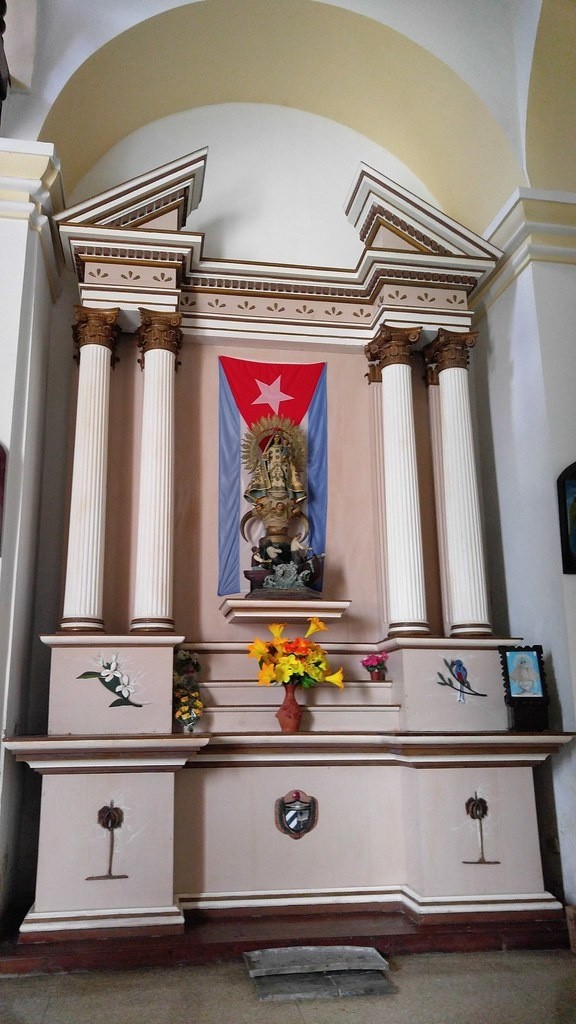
[497,644,551,707]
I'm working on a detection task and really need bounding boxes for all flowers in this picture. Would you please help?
[360,651,388,673]
[173,644,208,726]
[247,617,345,693]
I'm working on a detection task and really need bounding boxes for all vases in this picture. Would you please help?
[371,671,386,681]
[274,684,302,732]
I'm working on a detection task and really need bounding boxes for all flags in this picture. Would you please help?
[217,354,328,597]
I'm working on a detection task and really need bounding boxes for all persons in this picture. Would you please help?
[255,502,300,514]
[244,431,308,506]
[251,532,312,570]
